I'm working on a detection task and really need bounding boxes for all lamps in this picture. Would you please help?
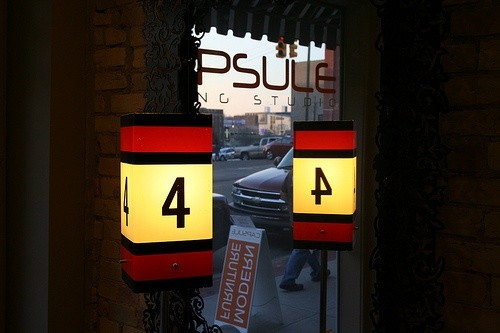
[120,112,214,333]
[292,120,360,333]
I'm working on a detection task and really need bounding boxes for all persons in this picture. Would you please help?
[279,171,331,291]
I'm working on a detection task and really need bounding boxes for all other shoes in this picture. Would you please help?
[311,269,331,282]
[279,282,304,291]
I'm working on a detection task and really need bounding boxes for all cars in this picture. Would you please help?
[212,191,235,252]
[227,146,294,246]
[211,134,294,161]
